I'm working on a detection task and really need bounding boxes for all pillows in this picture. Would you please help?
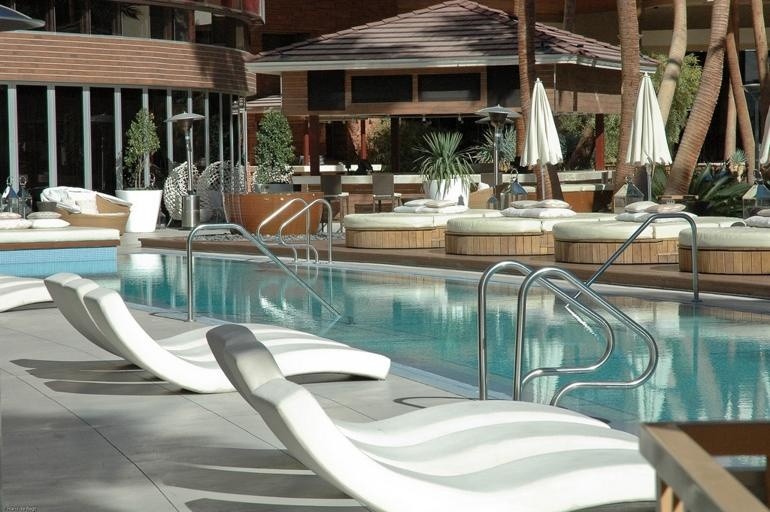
[67,190,99,214]
[758,209,770,217]
[0,211,23,219]
[392,198,470,214]
[32,219,71,228]
[613,199,699,225]
[497,200,576,218]
[743,216,770,228]
[26,210,62,219]
[0,219,32,229]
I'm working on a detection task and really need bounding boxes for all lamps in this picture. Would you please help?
[496,167,528,210]
[740,166,770,219]
[14,174,33,215]
[0,174,19,213]
[612,175,644,214]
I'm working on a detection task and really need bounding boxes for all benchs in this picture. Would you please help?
[345,209,483,256]
[546,214,739,270]
[442,211,613,261]
[668,223,770,279]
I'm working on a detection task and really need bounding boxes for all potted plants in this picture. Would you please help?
[112,107,166,233]
[249,105,300,192]
[414,129,479,205]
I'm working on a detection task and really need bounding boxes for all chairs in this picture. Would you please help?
[467,181,496,210]
[371,169,402,214]
[166,159,216,230]
[199,160,247,226]
[200,316,661,512]
[37,184,133,240]
[318,174,351,236]
[36,266,393,394]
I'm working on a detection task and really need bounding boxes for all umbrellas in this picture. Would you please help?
[624,72,673,201]
[519,77,564,200]
[758,105,770,164]
